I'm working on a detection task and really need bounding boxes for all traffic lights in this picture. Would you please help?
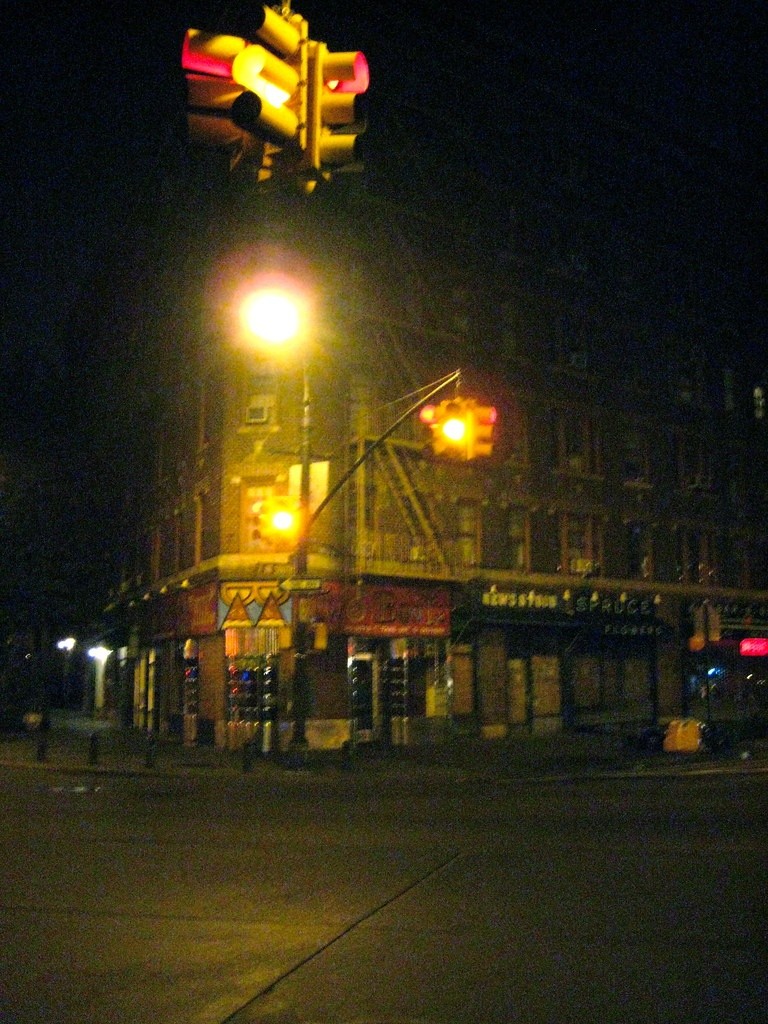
[468,403,499,461]
[421,400,445,459]
[439,403,468,462]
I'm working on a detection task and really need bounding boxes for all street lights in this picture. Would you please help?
[240,272,318,767]
